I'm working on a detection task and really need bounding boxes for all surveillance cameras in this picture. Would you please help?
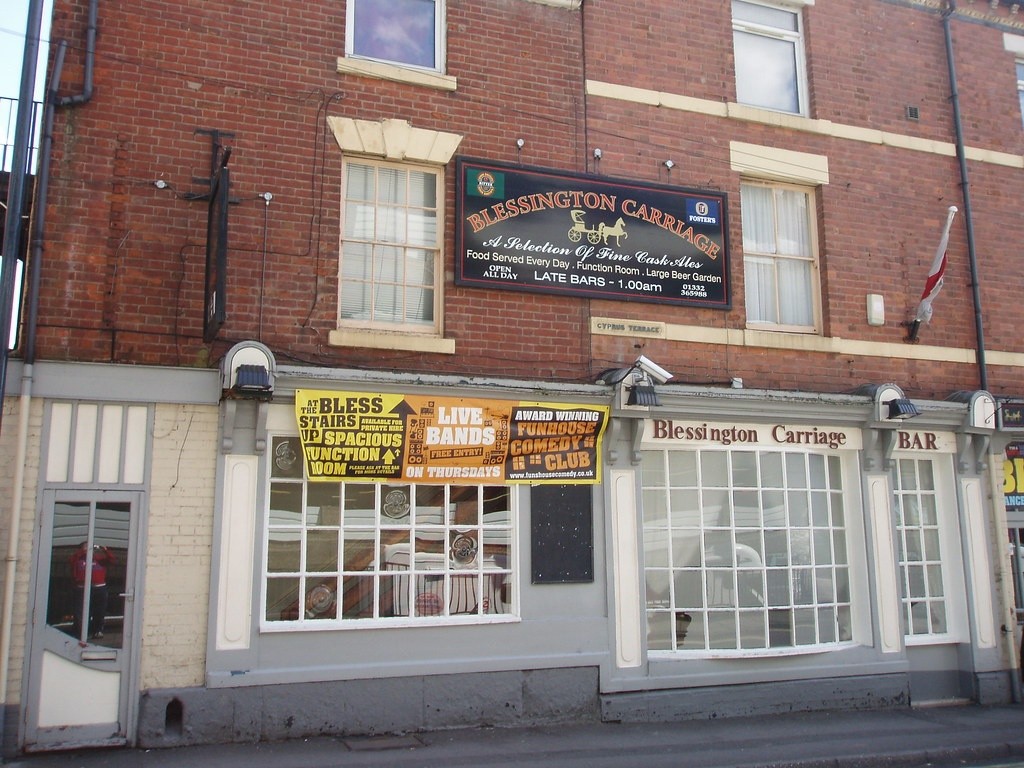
[635,355,674,383]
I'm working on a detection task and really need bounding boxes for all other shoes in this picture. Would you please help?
[91,632,103,639]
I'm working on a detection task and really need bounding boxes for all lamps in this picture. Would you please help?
[882,399,923,419]
[235,363,272,392]
[626,385,663,407]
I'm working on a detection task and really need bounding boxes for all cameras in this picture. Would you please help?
[93,545,99,549]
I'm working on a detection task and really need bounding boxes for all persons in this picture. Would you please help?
[70,541,118,639]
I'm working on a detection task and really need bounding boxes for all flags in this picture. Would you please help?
[917,229,948,326]
